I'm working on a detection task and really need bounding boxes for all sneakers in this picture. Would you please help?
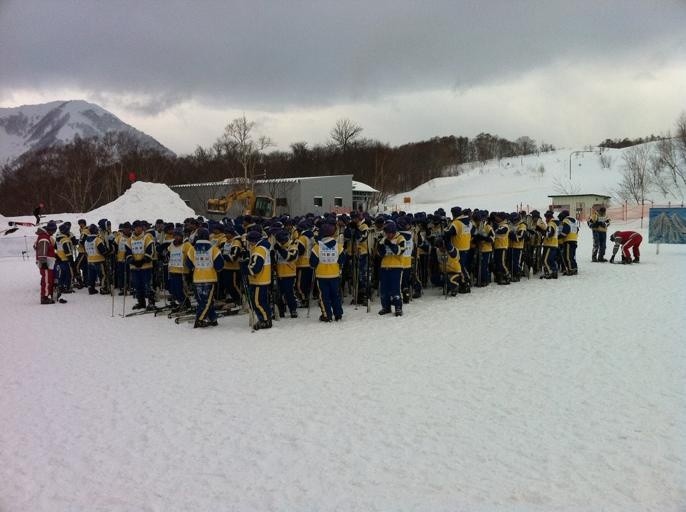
[379,289,410,316]
[41,297,54,304]
[61,282,98,294]
[132,302,156,310]
[474,275,519,287]
[290,298,308,317]
[413,288,421,298]
[618,257,640,264]
[253,320,271,330]
[540,269,577,279]
[197,320,217,328]
[592,255,607,262]
[119,288,135,296]
[320,315,342,322]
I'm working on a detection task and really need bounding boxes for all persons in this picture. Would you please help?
[33,203,44,225]
[36,206,580,331]
[610,231,642,264]
[592,207,608,262]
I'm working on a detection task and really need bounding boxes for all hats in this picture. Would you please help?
[119,216,209,240]
[451,207,570,223]
[224,209,445,242]
[45,218,110,234]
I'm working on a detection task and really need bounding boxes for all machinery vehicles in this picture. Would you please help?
[207,189,275,220]
